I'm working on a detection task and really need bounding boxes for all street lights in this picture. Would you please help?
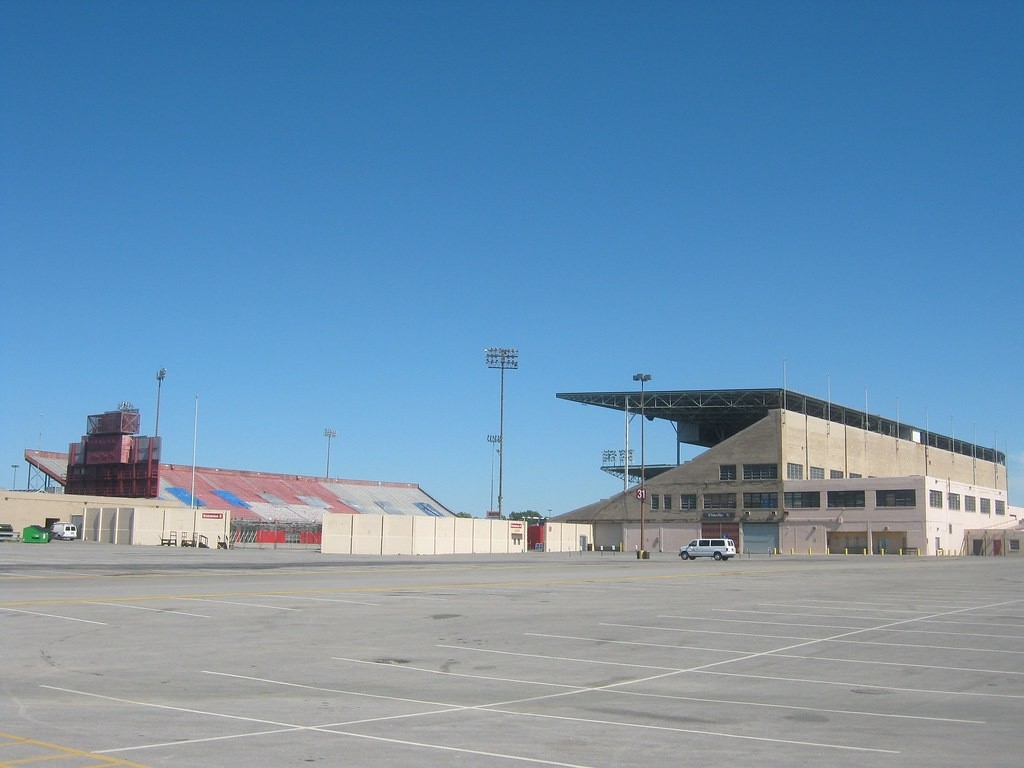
[35,413,44,491]
[155,368,168,436]
[485,434,502,511]
[632,373,651,560]
[484,346,520,519]
[324,428,337,479]
[189,394,199,512]
[11,465,19,491]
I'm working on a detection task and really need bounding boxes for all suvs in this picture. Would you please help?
[678,537,736,562]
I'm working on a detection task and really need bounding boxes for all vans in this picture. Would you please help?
[50,521,77,541]
